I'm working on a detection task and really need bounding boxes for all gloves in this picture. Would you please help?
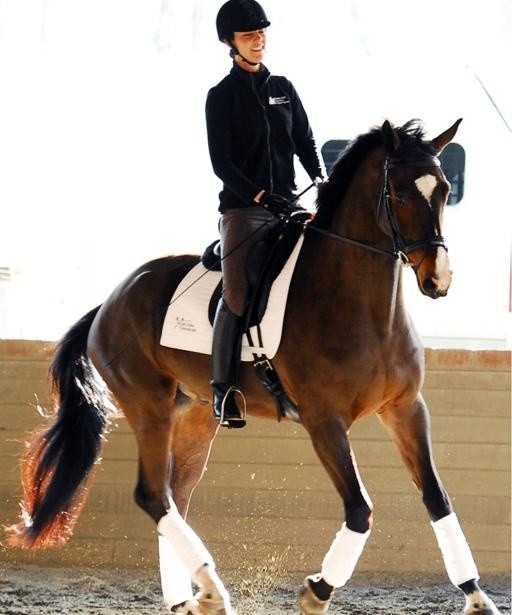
[257,191,296,222]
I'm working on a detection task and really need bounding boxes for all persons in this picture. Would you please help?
[204,0,333,429]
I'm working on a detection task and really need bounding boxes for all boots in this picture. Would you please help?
[209,298,248,418]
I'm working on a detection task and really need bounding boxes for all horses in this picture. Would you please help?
[0,114,512,615]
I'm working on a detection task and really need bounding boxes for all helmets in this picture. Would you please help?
[216,0,270,41]
